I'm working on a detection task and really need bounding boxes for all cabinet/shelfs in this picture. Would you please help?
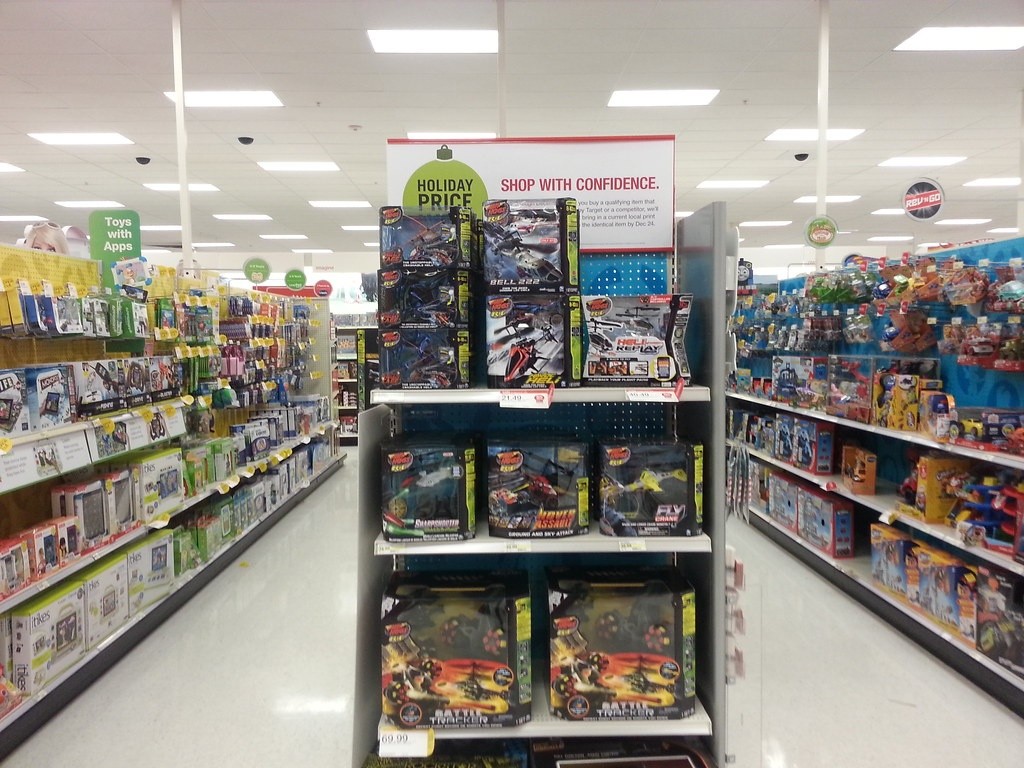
[371,388,713,741]
[337,326,378,438]
[0,288,347,764]
[725,390,1024,719]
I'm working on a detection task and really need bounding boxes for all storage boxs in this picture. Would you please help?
[378,328,475,389]
[362,739,528,768]
[581,293,692,387]
[485,432,589,539]
[548,571,696,721]
[378,268,476,328]
[593,433,703,537]
[482,198,580,295]
[750,354,1024,680]
[379,430,482,543]
[381,570,532,729]
[486,294,581,388]
[529,736,719,768]
[0,288,330,720]
[379,206,483,273]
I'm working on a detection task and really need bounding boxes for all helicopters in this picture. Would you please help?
[495,316,551,383]
